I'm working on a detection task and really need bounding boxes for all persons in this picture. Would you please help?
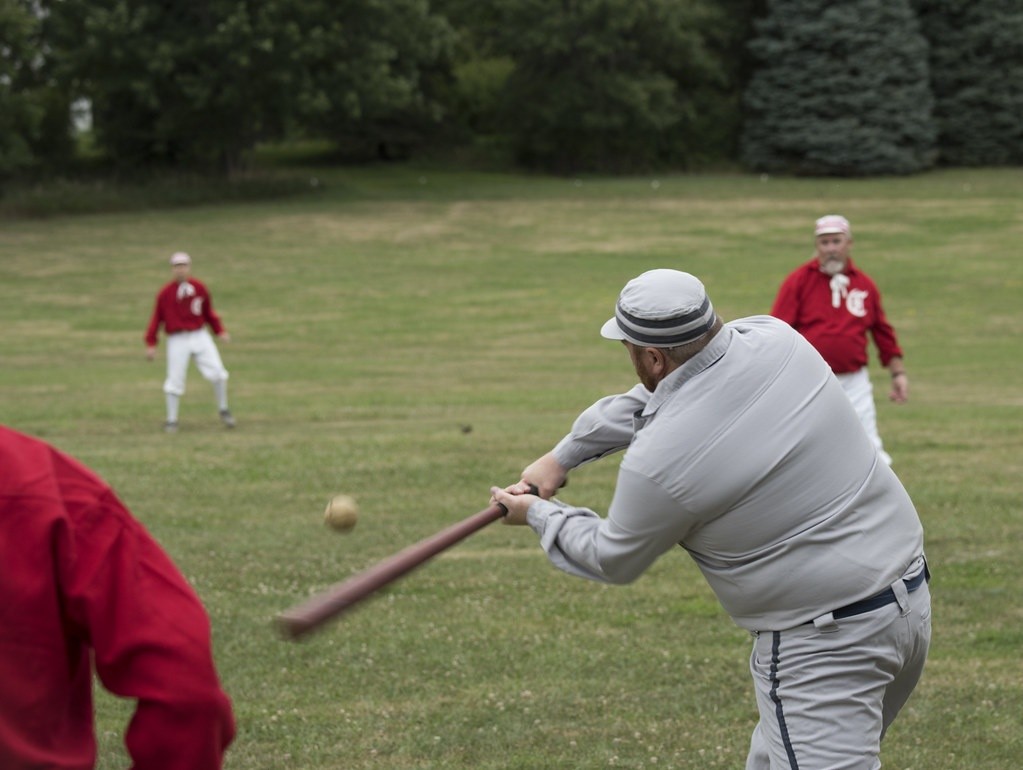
[0,425,236,770]
[491,269,933,770]
[771,213,909,468]
[145,251,237,433]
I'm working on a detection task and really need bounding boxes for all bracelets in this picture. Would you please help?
[893,370,904,377]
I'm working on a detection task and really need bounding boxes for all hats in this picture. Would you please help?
[599,267,716,348]
[170,251,190,266]
[813,215,851,241]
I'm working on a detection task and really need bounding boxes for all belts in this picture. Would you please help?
[801,566,925,626]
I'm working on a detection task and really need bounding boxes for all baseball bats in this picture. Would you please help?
[271,476,568,643]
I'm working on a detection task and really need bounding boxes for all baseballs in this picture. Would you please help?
[319,495,361,537]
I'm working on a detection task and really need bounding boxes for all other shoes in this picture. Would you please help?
[162,422,179,437]
[219,411,236,427]
